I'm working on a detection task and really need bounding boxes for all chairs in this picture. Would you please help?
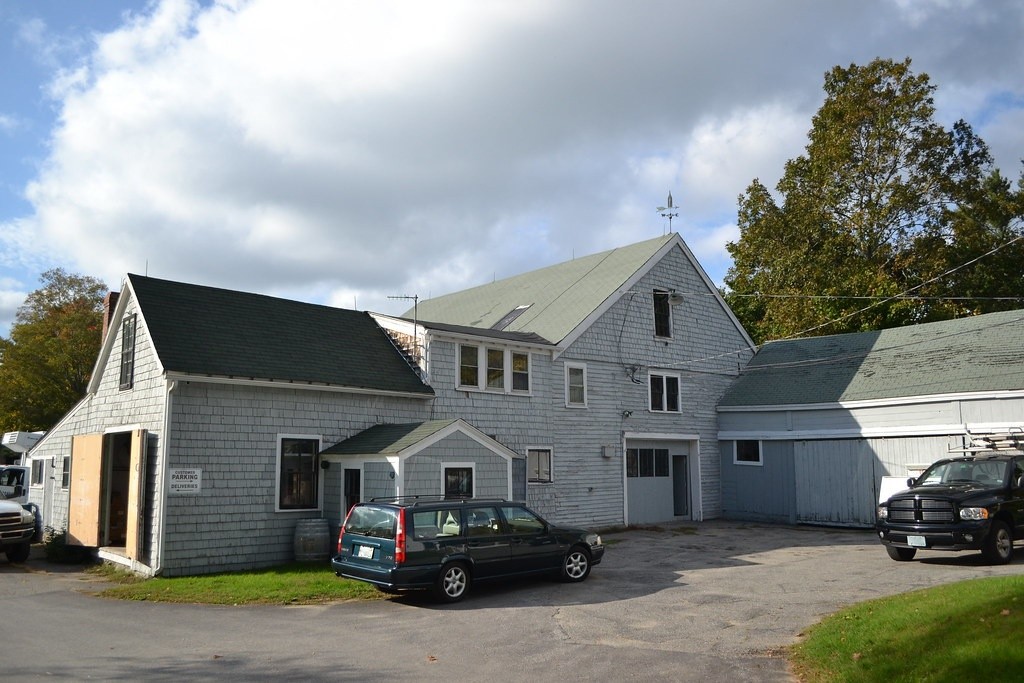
[468,511,493,536]
[442,512,461,536]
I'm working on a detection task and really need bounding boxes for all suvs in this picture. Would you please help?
[876,426,1024,565]
[329,492,605,604]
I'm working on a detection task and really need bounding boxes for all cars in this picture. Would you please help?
[0,488,35,565]
